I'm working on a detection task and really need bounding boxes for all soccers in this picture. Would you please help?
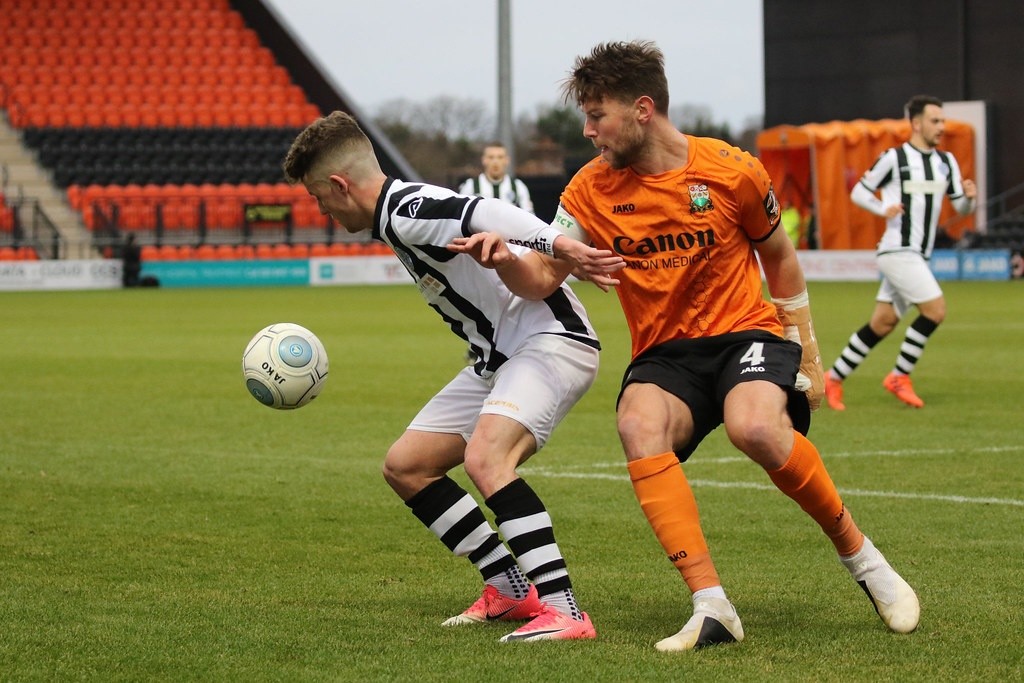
[240,322,332,411]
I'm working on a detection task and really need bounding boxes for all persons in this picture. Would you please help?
[458,144,536,216]
[446,41,921,651]
[822,94,978,409]
[284,111,626,647]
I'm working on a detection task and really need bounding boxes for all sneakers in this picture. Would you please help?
[851,548,920,633]
[500,601,595,641]
[882,371,926,408]
[440,583,541,625]
[823,370,846,412]
[654,601,744,651]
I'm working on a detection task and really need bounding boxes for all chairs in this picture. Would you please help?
[1,0,410,255]
